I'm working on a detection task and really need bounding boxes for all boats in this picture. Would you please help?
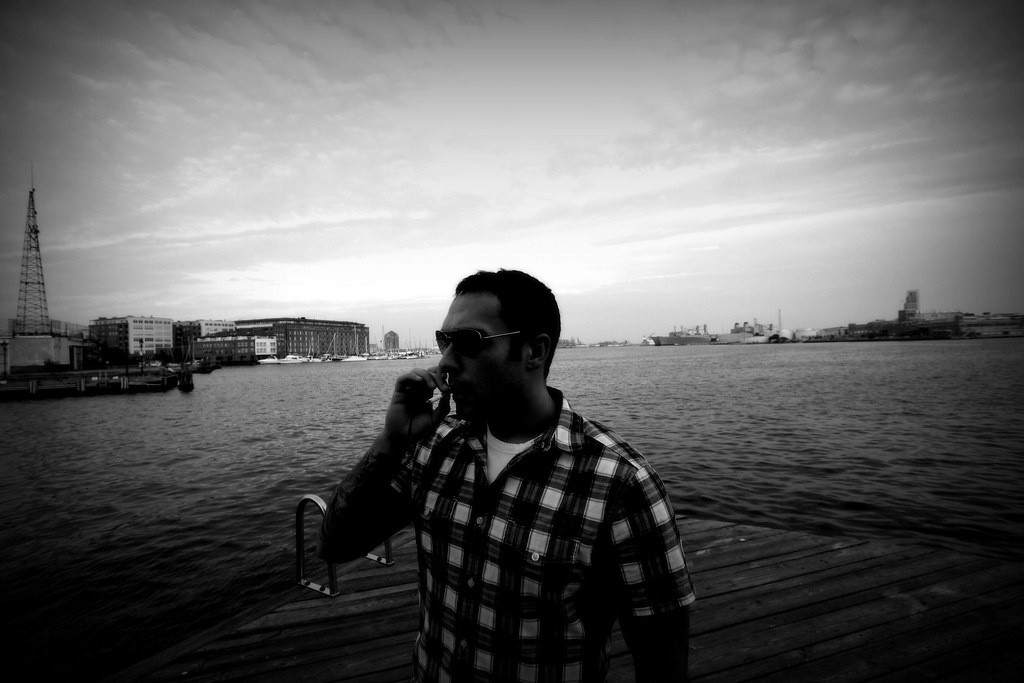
[651,324,769,346]
[257,333,425,364]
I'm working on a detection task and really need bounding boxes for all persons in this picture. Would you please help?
[316,269,694,683]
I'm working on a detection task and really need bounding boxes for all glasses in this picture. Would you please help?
[435,328,520,359]
